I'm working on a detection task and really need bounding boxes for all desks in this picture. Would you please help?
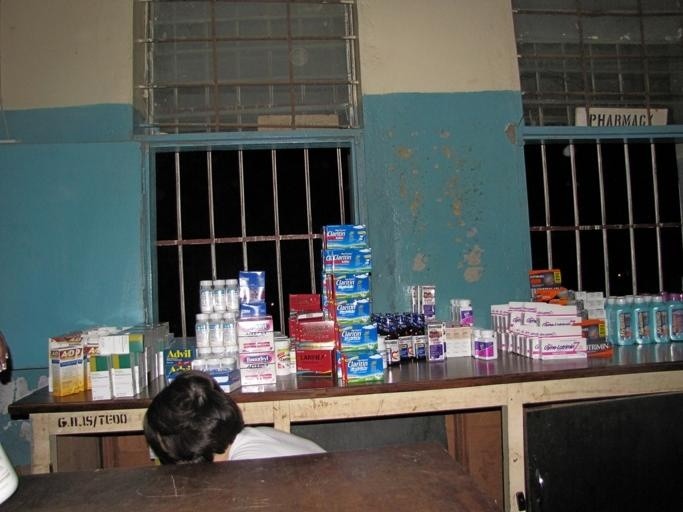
[0,440,502,511]
[7,340,682,511]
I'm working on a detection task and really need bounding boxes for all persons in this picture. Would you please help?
[143,369,328,466]
